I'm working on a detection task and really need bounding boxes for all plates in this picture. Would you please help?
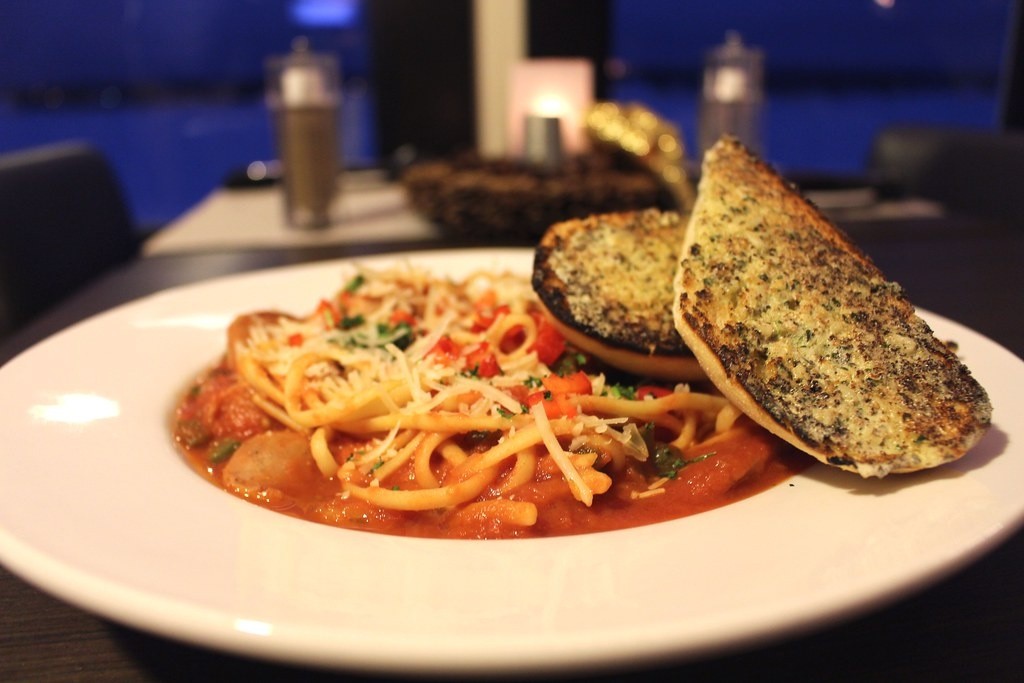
[0,246,1024,680]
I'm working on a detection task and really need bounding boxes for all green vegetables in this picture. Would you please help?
[317,270,716,478]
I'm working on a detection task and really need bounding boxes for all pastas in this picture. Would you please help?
[227,263,748,530]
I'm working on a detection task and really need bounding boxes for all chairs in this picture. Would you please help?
[0,140,145,344]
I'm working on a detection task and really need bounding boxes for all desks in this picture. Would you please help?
[0,164,1024,683]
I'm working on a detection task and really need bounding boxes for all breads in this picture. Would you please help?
[671,134,992,479]
[529,207,712,380]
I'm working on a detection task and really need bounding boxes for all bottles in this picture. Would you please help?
[276,67,341,229]
[700,29,765,167]
